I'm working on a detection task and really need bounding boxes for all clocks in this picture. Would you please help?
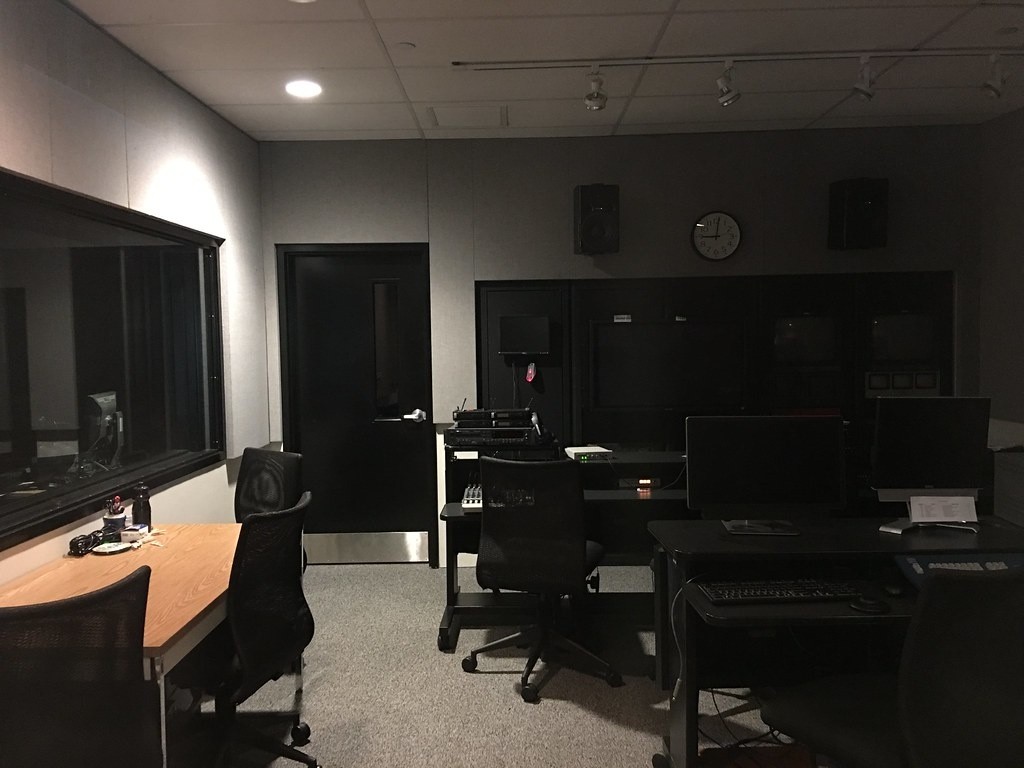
[690,210,743,261]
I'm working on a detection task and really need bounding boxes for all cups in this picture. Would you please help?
[103,515,126,529]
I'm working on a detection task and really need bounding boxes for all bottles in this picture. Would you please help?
[132,481,152,533]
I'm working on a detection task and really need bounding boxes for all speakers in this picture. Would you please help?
[828,175,889,251]
[573,183,620,253]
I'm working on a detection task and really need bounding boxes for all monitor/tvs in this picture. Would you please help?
[868,311,935,363]
[589,317,746,413]
[870,396,991,533]
[83,392,124,475]
[498,314,550,354]
[685,415,847,534]
[772,312,841,367]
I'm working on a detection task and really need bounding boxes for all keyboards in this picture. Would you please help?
[698,578,883,605]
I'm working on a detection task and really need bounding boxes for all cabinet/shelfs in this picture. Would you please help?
[475,269,957,565]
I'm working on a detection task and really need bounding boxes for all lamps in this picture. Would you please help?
[582,65,608,111]
[982,54,1012,101]
[853,55,879,103]
[716,59,743,109]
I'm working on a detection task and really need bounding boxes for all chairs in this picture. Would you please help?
[0,448,322,768]
[758,568,1024,768]
[462,455,619,700]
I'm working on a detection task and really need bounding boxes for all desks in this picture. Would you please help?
[437,445,1024,768]
[0,520,244,767]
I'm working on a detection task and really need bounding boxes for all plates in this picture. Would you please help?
[92,542,131,554]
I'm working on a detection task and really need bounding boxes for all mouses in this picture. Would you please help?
[885,581,906,598]
